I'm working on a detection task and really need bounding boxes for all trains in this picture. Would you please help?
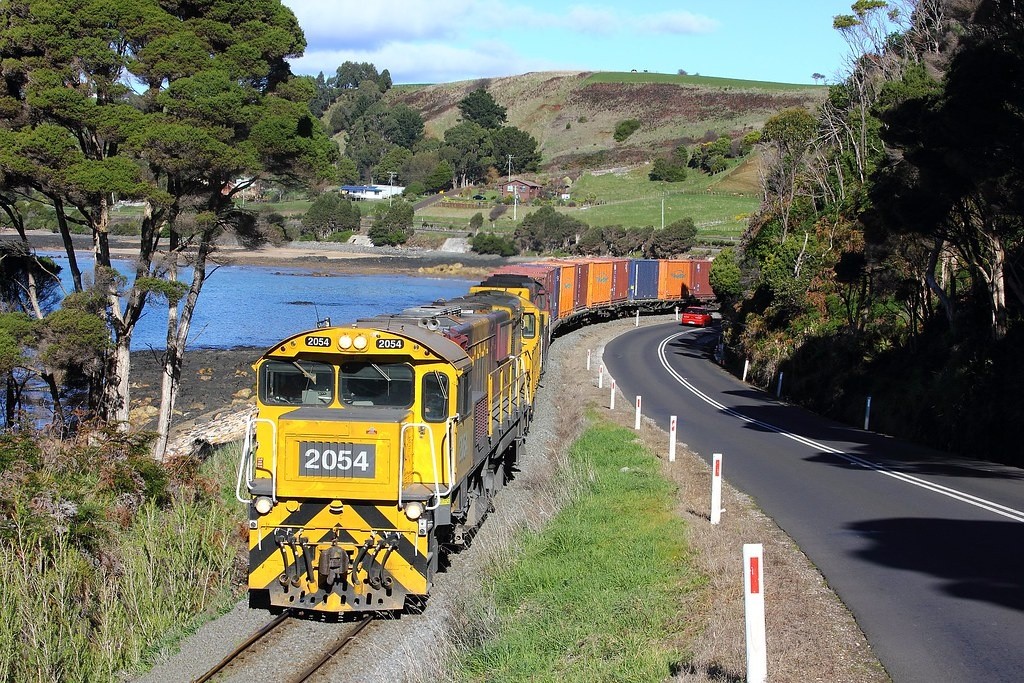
[233,258,722,617]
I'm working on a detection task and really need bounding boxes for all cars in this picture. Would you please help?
[681,307,714,327]
